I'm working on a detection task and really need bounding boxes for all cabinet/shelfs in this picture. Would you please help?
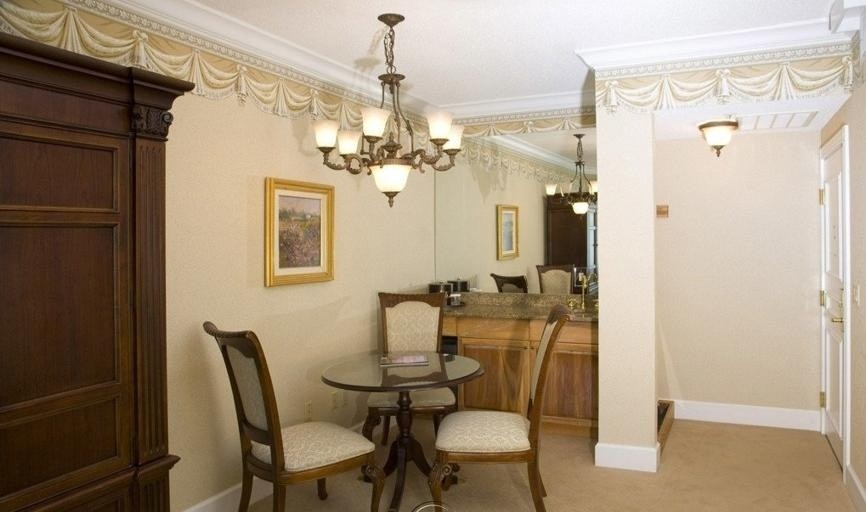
[1,32,195,512]
[454,316,598,438]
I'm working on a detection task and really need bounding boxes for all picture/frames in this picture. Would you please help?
[496,205,520,261]
[264,176,335,287]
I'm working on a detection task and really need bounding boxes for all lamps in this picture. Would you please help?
[314,13,464,208]
[546,134,596,222]
[691,108,744,157]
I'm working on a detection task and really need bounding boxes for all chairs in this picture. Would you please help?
[535,264,575,293]
[203,321,386,511]
[490,272,529,292]
[428,304,574,511]
[361,291,464,484]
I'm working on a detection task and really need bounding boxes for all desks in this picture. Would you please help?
[324,348,486,511]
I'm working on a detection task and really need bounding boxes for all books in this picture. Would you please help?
[379,354,429,367]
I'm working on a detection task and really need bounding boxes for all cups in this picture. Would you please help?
[450,293,461,306]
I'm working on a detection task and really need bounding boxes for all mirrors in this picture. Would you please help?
[428,107,602,295]
